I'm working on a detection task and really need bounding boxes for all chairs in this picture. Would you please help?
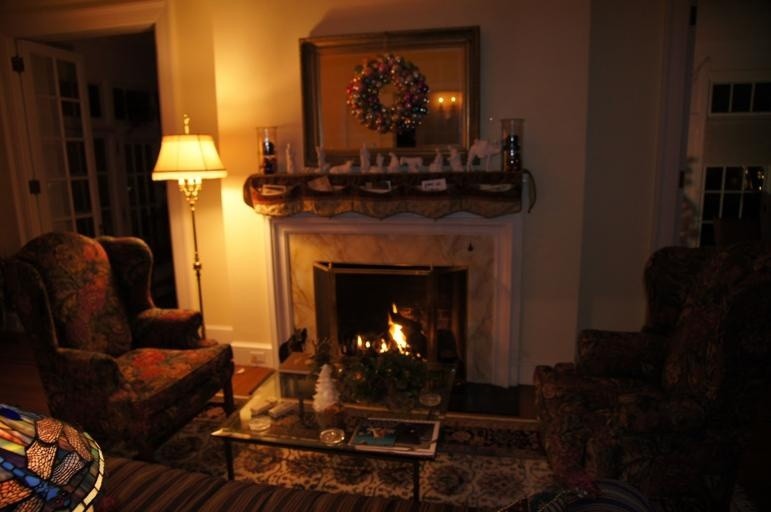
[0,229,235,462]
[533,241,771,512]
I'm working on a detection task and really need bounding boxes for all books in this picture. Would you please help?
[346,416,441,455]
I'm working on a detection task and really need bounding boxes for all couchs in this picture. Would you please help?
[96,457,483,512]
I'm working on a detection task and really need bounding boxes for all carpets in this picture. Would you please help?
[135,390,562,512]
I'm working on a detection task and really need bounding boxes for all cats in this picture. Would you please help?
[278,326,308,363]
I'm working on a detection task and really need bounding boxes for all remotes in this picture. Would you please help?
[250,399,276,415]
[269,400,298,418]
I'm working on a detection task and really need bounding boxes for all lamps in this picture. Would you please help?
[0,403,106,512]
[149,112,229,341]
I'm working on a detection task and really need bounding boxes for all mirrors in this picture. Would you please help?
[298,24,481,170]
